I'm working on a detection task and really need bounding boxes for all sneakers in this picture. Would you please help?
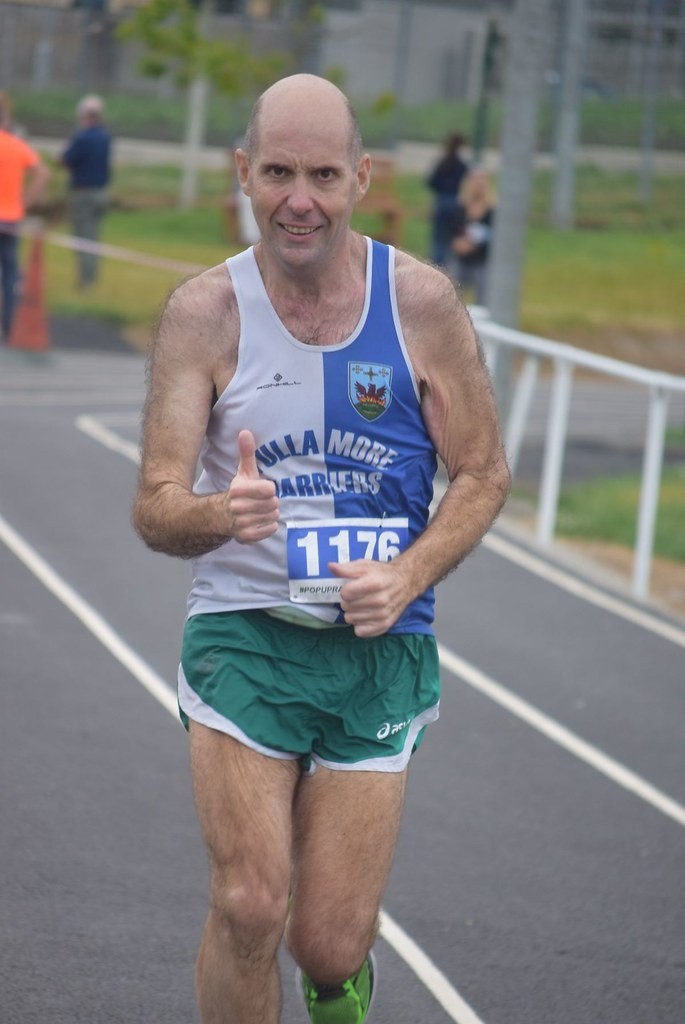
[287,889,377,1024]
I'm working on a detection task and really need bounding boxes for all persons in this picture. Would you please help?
[135,74,512,1023]
[0,95,110,333]
[428,129,500,298]
